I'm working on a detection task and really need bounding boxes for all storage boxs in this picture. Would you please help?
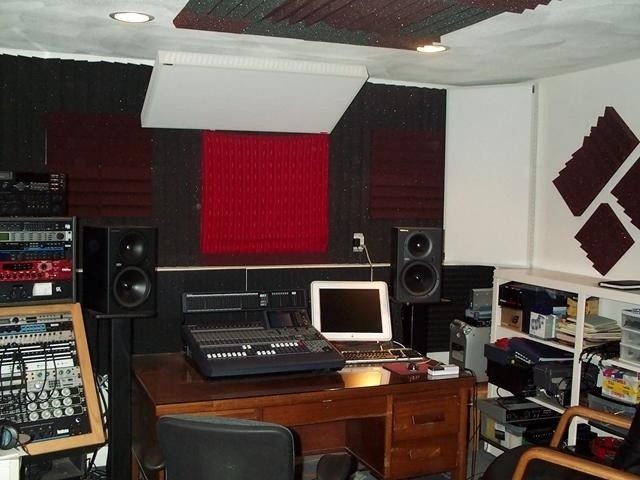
[618,303,640,370]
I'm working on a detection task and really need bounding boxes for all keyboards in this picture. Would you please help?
[340,348,426,364]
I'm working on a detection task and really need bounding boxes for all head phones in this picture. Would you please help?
[1,419,34,449]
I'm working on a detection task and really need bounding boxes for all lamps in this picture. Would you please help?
[351,233,376,281]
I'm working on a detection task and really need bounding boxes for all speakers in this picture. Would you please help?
[390,226,444,305]
[80,224,159,314]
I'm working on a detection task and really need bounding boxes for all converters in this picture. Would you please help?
[353,238,360,247]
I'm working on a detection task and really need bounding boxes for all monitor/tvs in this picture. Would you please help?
[310,281,393,341]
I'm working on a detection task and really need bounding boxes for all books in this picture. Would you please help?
[555,314,621,346]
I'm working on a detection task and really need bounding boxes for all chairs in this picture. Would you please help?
[478,386,640,479]
[130,404,358,479]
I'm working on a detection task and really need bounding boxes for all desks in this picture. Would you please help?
[126,341,474,479]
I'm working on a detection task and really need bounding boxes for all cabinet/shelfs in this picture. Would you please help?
[482,264,640,460]
